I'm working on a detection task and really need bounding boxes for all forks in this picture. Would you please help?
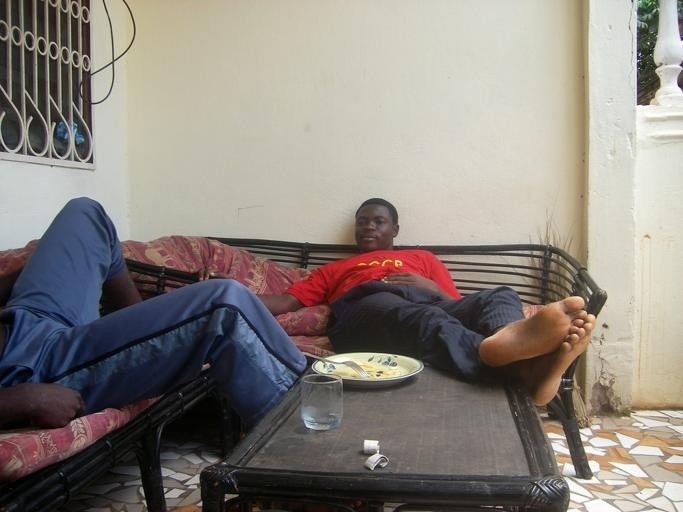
[301,351,367,378]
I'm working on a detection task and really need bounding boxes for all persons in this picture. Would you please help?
[255,197,597,409]
[0,195,309,431]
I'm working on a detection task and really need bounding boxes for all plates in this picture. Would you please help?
[311,351,425,390]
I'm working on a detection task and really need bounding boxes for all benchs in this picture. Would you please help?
[201,237,607,481]
[0,257,230,512]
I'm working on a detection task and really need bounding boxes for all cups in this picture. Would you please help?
[299,373,344,432]
